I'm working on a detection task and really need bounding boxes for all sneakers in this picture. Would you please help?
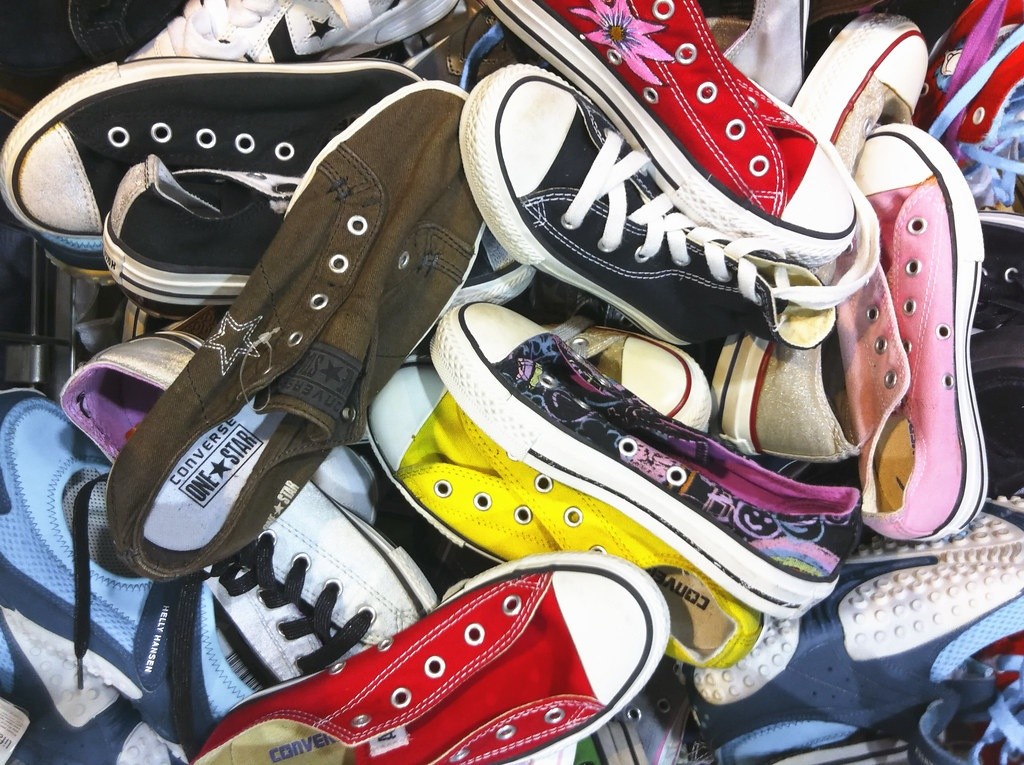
[1,0,1024,765]
